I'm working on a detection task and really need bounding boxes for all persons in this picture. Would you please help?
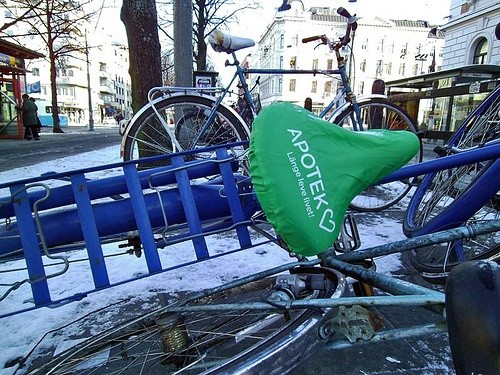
[15,93,39,139]
[29,96,43,134]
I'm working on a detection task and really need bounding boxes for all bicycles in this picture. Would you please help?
[117,7,424,213]
[0,84,500,375]
